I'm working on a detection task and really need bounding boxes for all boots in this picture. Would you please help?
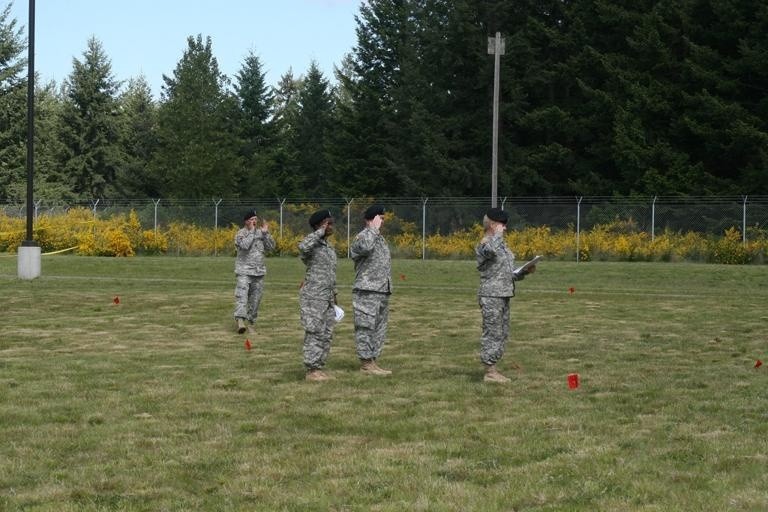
[484,365,511,383]
[247,321,259,335]
[306,368,338,380]
[237,318,246,334]
[360,357,392,376]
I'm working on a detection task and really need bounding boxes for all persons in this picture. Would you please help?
[348,204,396,378]
[294,208,345,384]
[474,206,537,386]
[231,208,278,336]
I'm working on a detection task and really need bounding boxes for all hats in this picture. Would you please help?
[363,205,386,218]
[310,209,332,227]
[486,208,509,224]
[243,210,258,221]
